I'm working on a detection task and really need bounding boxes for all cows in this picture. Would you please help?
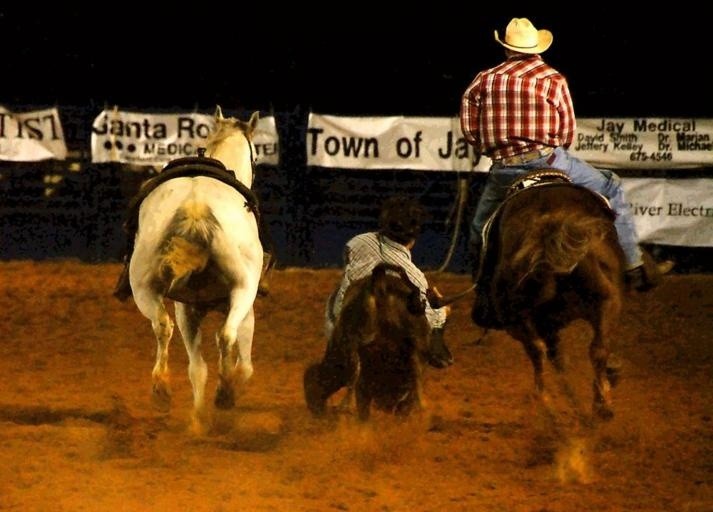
[302,262,477,423]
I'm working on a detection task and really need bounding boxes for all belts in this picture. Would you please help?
[493,146,553,166]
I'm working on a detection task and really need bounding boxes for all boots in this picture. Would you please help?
[424,332,453,366]
[624,260,676,293]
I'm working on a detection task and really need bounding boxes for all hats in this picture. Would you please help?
[494,18,554,54]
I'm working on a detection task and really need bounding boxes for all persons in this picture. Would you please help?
[460,17,675,292]
[305,197,449,419]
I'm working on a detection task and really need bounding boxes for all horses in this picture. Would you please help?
[128,105,265,442]
[488,183,631,423]
